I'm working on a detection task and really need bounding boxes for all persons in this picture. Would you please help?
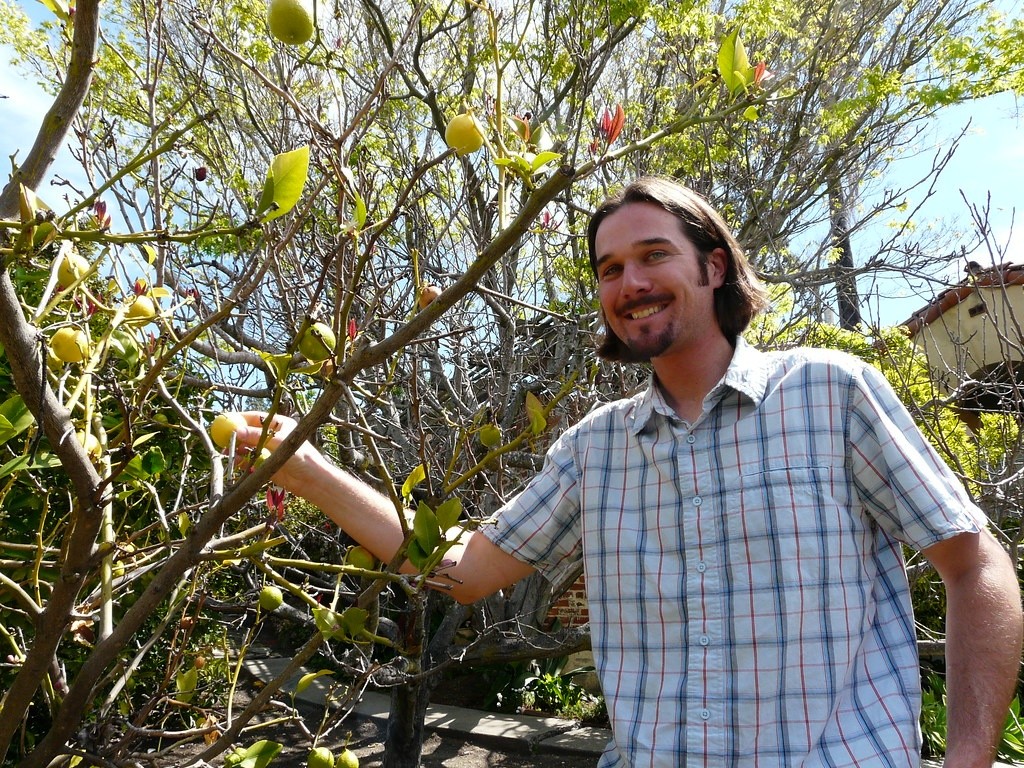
[221,174,1024,768]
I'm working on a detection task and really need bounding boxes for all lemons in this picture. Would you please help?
[0,1,502,768]
